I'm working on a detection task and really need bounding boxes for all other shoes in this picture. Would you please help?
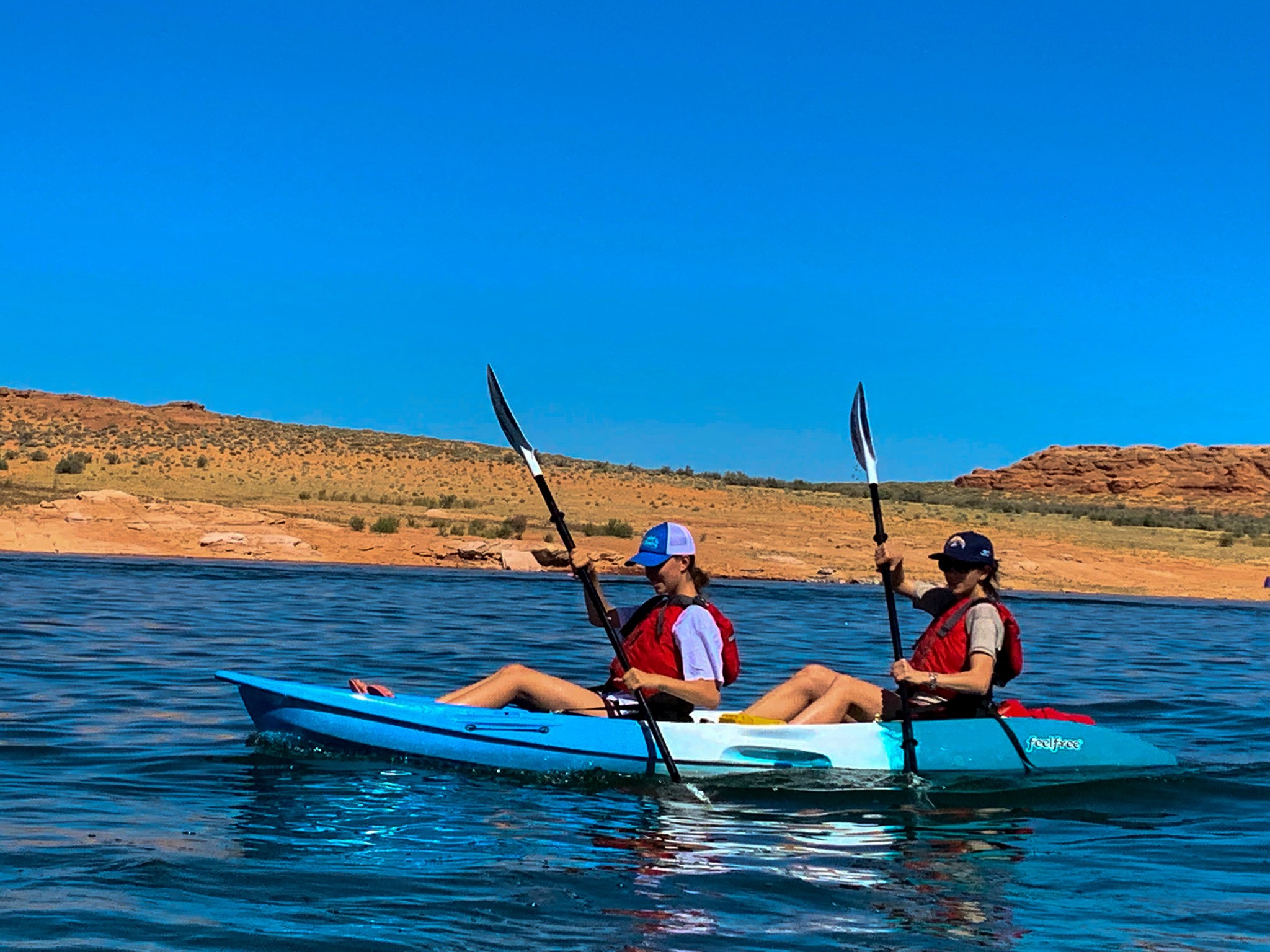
[349,679,368,693]
[367,685,394,698]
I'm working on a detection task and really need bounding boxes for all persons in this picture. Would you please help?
[350,521,739,720]
[700,531,1023,724]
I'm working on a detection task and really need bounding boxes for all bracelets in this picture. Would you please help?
[927,672,937,689]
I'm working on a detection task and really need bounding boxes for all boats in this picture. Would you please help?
[203,664,1186,788]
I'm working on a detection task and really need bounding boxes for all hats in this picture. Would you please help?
[624,522,696,568]
[928,530,994,562]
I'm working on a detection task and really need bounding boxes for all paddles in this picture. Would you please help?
[849,382,918,776]
[486,363,711,805]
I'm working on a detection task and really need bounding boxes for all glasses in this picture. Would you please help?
[938,558,985,574]
